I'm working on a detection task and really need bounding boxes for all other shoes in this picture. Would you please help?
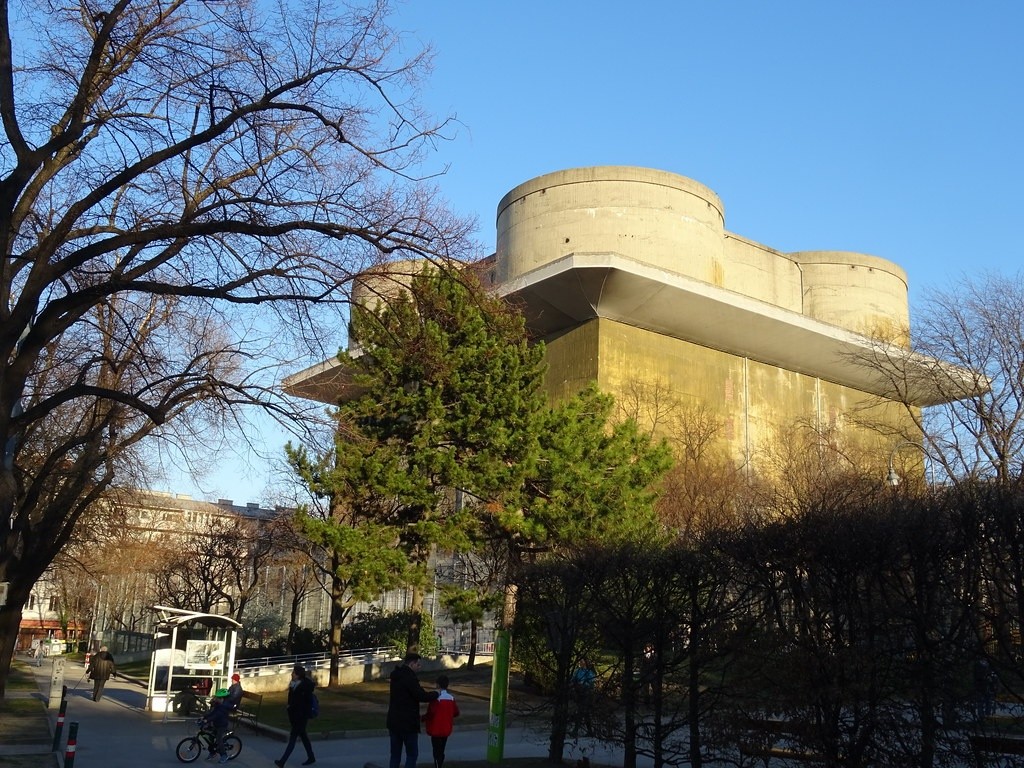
[207,751,217,760]
[302,757,315,765]
[275,760,284,768]
[218,752,229,763]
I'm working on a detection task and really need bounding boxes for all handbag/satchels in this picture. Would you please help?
[309,693,319,719]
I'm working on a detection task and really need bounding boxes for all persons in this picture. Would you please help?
[178,671,212,715]
[386,653,442,768]
[85,646,117,702]
[223,674,243,713]
[274,663,316,768]
[422,674,460,768]
[568,656,596,736]
[199,688,230,763]
[34,640,46,667]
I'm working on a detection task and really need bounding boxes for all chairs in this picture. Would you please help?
[181,679,213,716]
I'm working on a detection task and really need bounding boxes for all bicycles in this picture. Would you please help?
[175,716,243,763]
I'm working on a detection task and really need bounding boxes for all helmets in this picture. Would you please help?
[214,688,231,697]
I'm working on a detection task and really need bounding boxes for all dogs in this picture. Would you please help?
[62,685,67,699]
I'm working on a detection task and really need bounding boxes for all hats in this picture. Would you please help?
[231,674,240,682]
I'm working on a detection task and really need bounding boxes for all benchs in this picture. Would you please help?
[228,690,263,732]
